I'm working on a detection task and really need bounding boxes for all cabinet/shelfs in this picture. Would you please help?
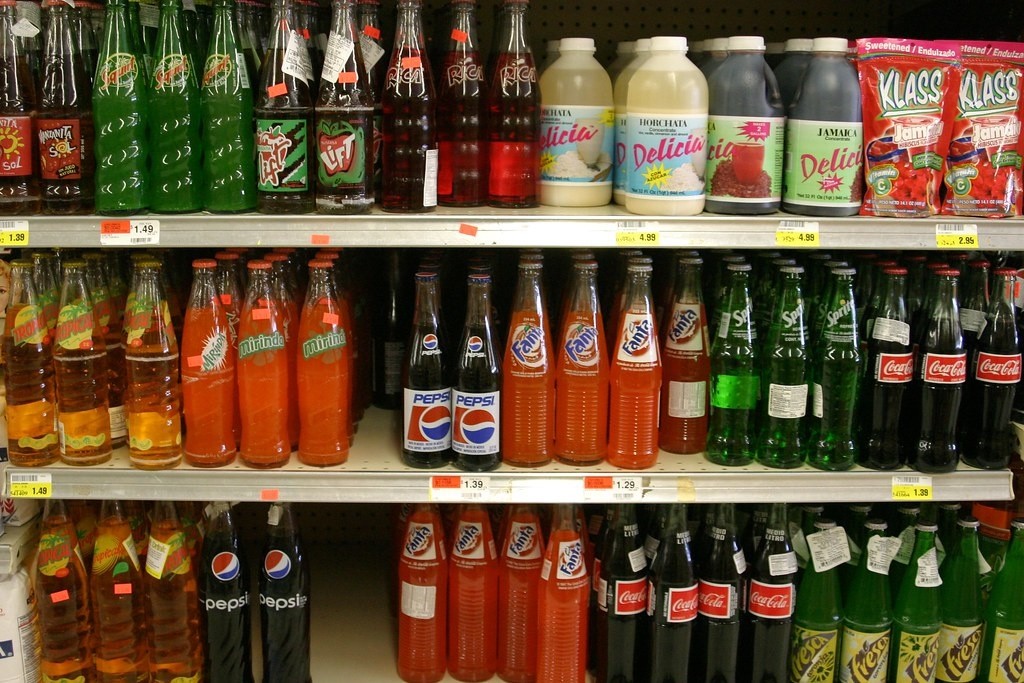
[0,204,1024,683]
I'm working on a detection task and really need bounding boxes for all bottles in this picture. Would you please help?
[0,499,315,683]
[0,0,538,215]
[539,36,863,217]
[3,249,361,470]
[396,503,1024,683]
[372,253,1023,469]
